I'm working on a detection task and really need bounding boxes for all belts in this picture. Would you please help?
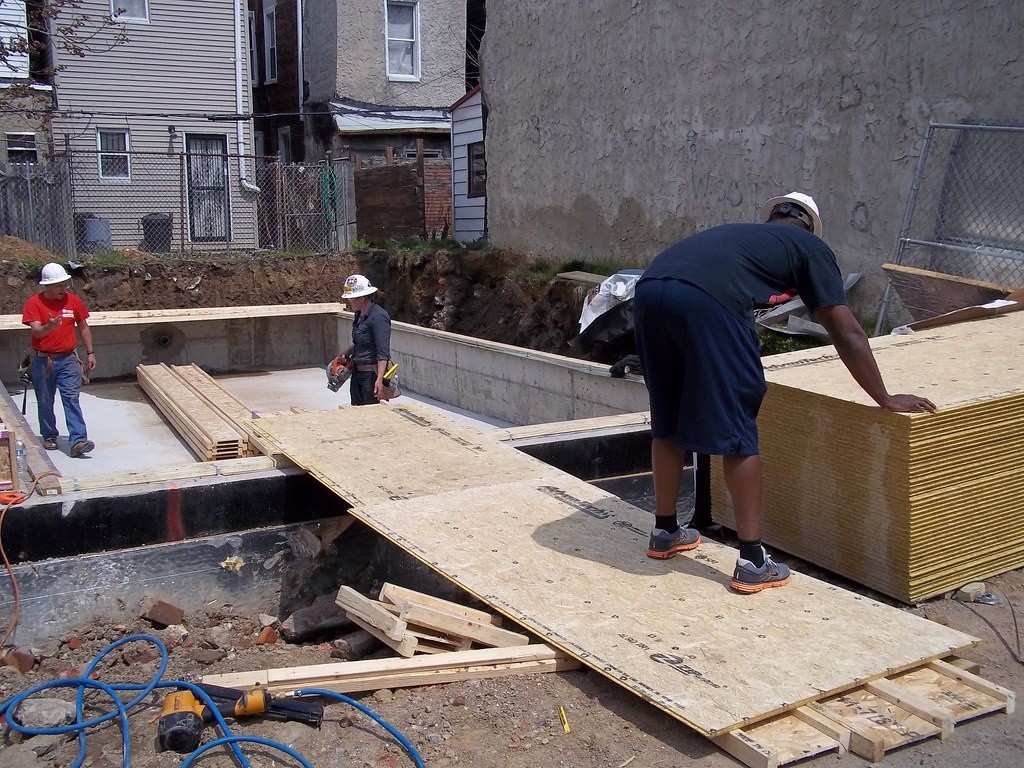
[37,350,72,379]
[352,364,374,371]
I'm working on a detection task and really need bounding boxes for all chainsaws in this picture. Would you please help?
[325,355,354,392]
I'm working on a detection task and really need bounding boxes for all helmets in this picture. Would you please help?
[341,274,377,298]
[38,262,72,285]
[758,192,824,237]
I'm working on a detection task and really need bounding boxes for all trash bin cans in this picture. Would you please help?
[142,212,173,251]
[85,217,111,254]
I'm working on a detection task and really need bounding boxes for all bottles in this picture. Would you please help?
[389,375,398,388]
[15,435,28,479]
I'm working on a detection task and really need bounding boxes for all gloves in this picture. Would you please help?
[609,355,642,379]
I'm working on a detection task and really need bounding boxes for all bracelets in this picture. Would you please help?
[87,349,94,354]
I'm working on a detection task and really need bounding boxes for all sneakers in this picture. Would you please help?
[71,440,95,458]
[648,523,701,559]
[730,546,791,593]
[45,438,57,450]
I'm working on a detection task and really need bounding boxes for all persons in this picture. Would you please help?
[342,275,391,406]
[634,193,937,593]
[22,263,96,457]
